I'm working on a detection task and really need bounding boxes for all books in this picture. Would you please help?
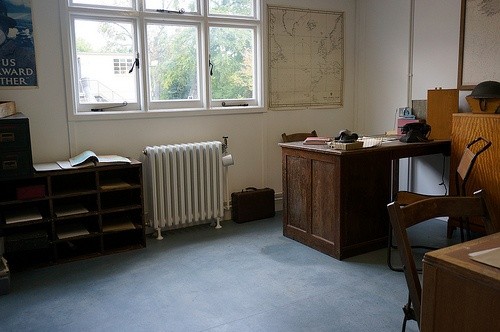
[303,137,331,145]
[56,151,131,170]
[329,142,364,150]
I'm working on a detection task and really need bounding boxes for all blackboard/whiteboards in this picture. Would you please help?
[457,0,500,90]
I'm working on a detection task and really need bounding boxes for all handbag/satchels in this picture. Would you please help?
[231,187,275,223]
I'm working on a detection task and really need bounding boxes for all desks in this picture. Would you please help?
[419,232,500,332]
[278,133,452,261]
[447,112,500,240]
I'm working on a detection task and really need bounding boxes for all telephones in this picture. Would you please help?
[401,123,432,142]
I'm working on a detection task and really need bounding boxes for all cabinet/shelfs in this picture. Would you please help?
[0,111,146,271]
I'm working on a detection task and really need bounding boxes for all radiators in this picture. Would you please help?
[142,136,234,241]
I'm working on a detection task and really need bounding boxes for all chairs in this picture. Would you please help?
[385,136,493,273]
[385,188,500,332]
[282,130,318,143]
